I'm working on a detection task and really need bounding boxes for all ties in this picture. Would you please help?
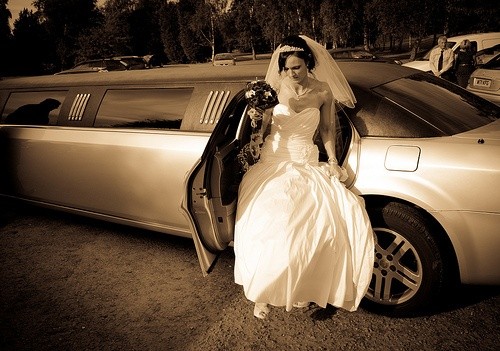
[438,50,444,70]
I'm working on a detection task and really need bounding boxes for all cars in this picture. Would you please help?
[54,54,163,76]
[439,44,500,106]
[210,53,236,68]
[330,50,376,59]
[0,61,500,319]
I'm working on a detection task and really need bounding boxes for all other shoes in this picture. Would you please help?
[254,303,268,319]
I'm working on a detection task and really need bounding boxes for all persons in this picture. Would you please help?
[410,39,420,61]
[4,98,61,124]
[454,40,475,87]
[429,36,454,79]
[234,36,375,320]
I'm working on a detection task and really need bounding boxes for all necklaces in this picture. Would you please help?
[290,77,309,101]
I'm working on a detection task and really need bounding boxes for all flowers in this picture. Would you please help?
[235,77,280,174]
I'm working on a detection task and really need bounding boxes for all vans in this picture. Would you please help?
[401,32,500,76]
